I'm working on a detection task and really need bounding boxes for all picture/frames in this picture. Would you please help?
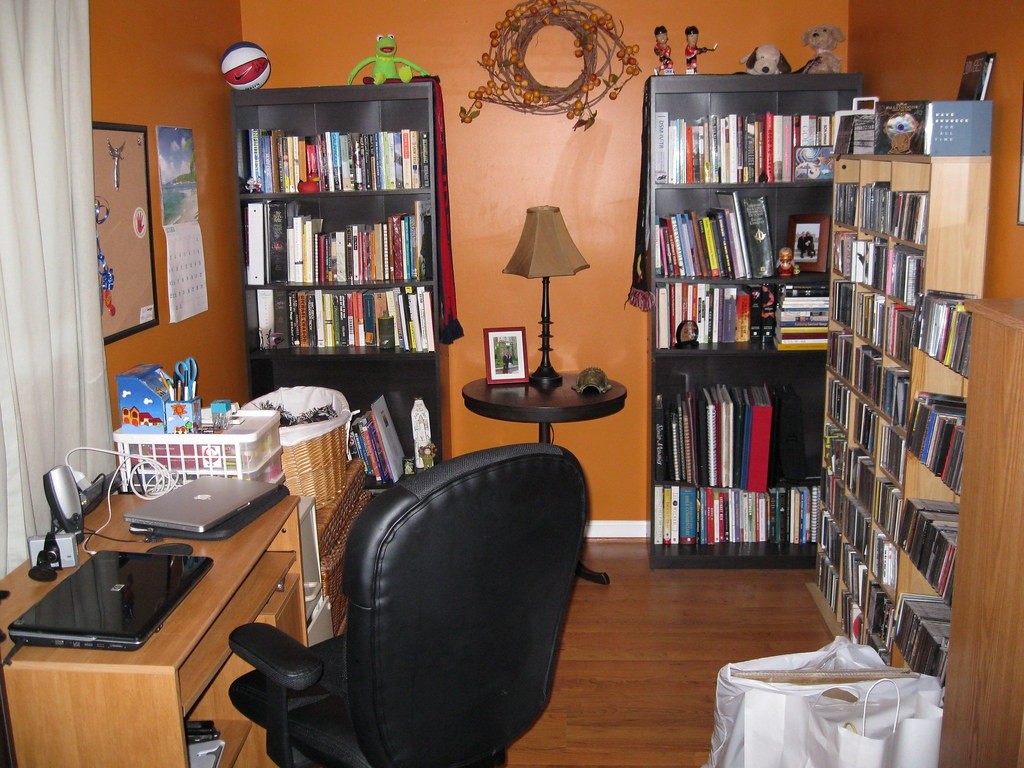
[92,120,161,346]
[482,326,530,385]
[784,213,831,273]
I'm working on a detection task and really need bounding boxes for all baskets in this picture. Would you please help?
[241,385,351,507]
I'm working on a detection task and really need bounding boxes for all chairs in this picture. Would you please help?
[223,443,592,768]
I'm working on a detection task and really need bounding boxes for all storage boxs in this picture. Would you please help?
[112,407,287,493]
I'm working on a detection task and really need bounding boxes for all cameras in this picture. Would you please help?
[28,533,79,567]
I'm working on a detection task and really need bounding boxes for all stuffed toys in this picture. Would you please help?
[801,23,847,74]
[740,45,792,75]
[347,34,429,85]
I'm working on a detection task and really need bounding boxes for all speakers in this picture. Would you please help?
[42,466,85,545]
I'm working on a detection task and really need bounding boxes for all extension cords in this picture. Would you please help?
[80,470,122,511]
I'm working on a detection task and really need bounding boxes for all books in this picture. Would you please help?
[654,384,822,545]
[346,395,406,484]
[249,129,430,192]
[817,181,977,710]
[656,283,828,350]
[957,50,996,100]
[245,286,435,352]
[654,112,836,184]
[245,199,433,285]
[655,190,776,280]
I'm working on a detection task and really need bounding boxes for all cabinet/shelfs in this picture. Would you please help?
[802,156,993,683]
[0,486,309,768]
[640,72,866,575]
[228,76,444,470]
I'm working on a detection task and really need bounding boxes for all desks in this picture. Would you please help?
[461,373,628,587]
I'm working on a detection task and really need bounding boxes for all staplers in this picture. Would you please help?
[187,720,219,745]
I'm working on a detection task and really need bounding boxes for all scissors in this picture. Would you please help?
[175,357,197,400]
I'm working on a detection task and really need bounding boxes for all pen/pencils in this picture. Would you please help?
[167,379,197,401]
[159,371,169,389]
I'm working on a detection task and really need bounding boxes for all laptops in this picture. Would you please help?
[8,550,214,650]
[123,475,278,531]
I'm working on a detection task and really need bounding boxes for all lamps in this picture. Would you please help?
[499,205,591,384]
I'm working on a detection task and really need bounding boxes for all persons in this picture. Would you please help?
[654,25,675,76]
[418,443,437,471]
[246,176,262,193]
[797,231,815,258]
[502,351,512,374]
[685,26,718,74]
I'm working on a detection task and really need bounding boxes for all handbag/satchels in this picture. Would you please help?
[701,635,943,768]
